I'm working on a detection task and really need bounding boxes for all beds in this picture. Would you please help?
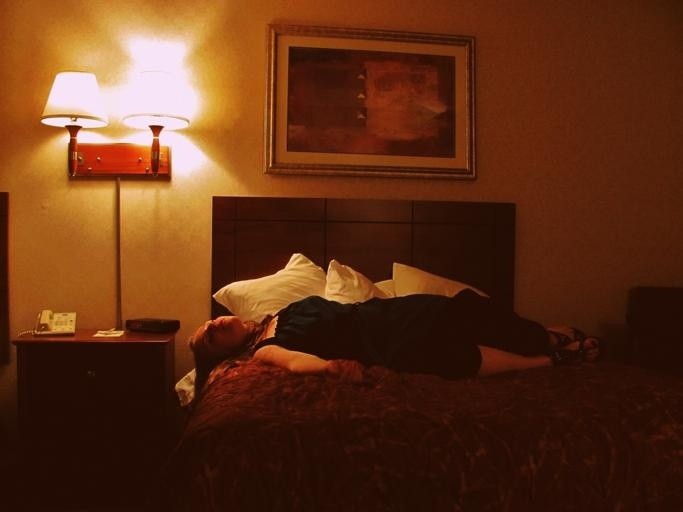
[187,195,683,511]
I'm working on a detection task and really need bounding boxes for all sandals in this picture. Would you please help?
[546,327,601,366]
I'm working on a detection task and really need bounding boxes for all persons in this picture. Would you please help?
[186,288,603,395]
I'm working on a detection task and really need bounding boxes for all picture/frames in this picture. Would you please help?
[263,21,479,183]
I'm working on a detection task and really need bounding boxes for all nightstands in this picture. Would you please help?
[12,329,177,511]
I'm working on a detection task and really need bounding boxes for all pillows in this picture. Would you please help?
[210,252,491,328]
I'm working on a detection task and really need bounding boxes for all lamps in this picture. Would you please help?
[38,69,191,180]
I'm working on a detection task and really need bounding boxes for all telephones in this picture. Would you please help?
[34,310,76,336]
